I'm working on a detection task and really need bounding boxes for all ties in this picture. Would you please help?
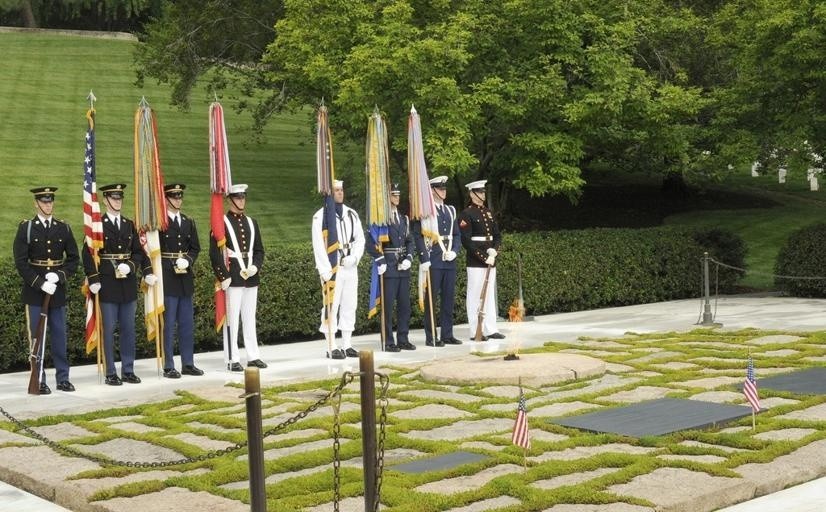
[440,205,444,212]
[45,220,50,230]
[174,216,179,227]
[394,212,399,224]
[114,217,118,230]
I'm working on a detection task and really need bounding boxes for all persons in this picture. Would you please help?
[312,179,366,360]
[209,183,268,371]
[366,183,416,352]
[14,187,80,394]
[141,184,204,379]
[413,175,462,348]
[82,184,144,386]
[458,180,505,342]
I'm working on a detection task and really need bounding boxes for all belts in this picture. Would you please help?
[232,252,249,259]
[31,259,64,266]
[341,244,351,249]
[383,247,406,254]
[441,236,450,241]
[161,253,187,259]
[471,236,494,241]
[100,254,131,260]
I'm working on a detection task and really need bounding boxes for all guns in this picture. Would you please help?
[29,294,51,395]
[475,264,491,341]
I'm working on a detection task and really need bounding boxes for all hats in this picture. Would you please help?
[30,187,58,202]
[99,184,127,199]
[229,184,248,197]
[164,184,185,198]
[391,183,401,196]
[335,179,343,187]
[465,180,488,193]
[430,176,448,190]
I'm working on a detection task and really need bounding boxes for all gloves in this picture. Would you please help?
[322,273,332,281]
[144,274,157,286]
[89,282,101,294]
[118,263,130,275]
[344,255,356,270]
[41,281,57,295]
[44,271,60,283]
[445,251,457,261]
[486,248,497,257]
[176,258,188,270]
[378,264,386,275]
[222,277,232,291]
[401,259,412,270]
[421,262,431,271]
[246,265,258,278]
[485,256,495,266]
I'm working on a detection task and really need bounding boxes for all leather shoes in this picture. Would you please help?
[121,373,141,383]
[181,366,204,375]
[247,359,267,368]
[39,382,51,394]
[105,374,122,385]
[327,333,506,359]
[227,362,244,371]
[163,368,181,378]
[56,381,75,391]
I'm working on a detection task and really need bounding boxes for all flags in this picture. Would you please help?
[208,103,233,334]
[83,109,105,356]
[512,390,531,450]
[407,115,437,313]
[317,106,339,324]
[364,113,393,319]
[743,357,761,412]
[134,106,168,342]
